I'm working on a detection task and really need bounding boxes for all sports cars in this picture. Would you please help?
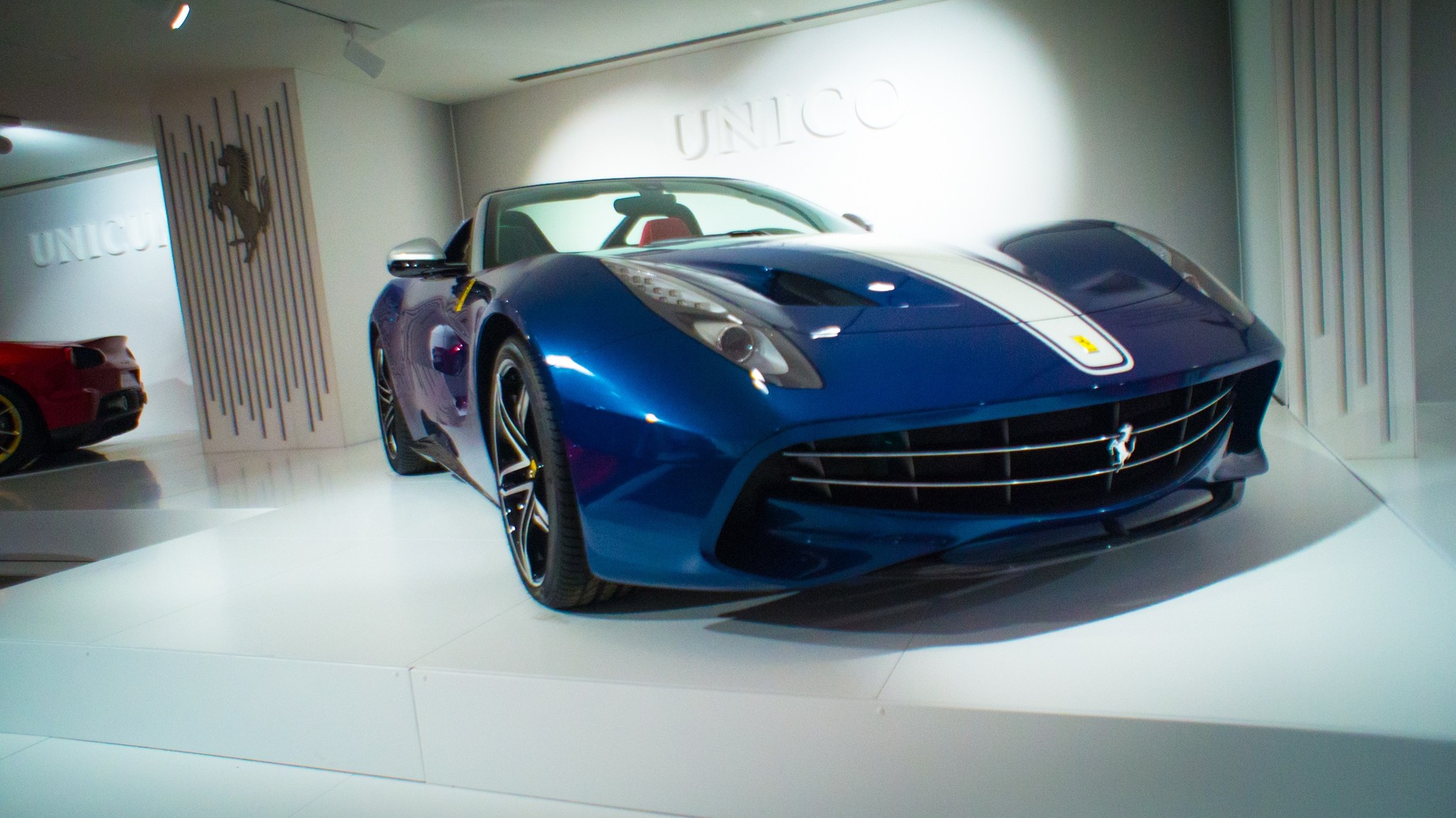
[367,173,1288,611]
[0,333,146,476]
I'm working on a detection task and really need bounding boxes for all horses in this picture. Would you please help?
[1108,422,1137,473]
[208,145,271,263]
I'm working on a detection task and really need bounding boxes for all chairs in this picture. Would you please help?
[441,209,560,268]
[638,219,693,247]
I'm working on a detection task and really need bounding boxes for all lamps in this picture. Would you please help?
[342,20,386,78]
[148,0,190,30]
